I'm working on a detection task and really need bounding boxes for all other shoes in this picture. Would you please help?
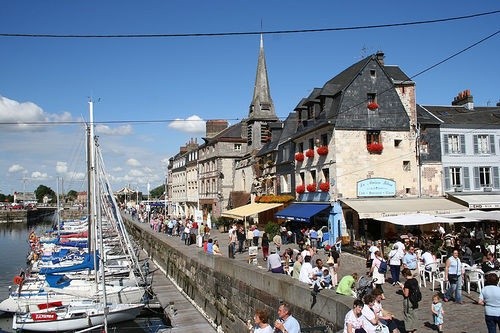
[438,330,443,333]
[392,282,395,286]
[443,294,448,302]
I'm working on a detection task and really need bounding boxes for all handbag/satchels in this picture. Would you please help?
[449,274,458,282]
[327,252,334,265]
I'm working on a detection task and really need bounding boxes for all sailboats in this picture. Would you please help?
[1,95,166,333]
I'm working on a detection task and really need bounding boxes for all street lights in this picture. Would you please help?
[165,176,168,220]
[123,185,127,213]
[136,186,138,216]
[147,182,150,225]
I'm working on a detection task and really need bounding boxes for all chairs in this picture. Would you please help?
[332,240,342,253]
[418,244,500,296]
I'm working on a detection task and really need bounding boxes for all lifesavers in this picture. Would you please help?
[15,276,23,284]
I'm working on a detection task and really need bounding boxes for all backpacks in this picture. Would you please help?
[375,257,387,274]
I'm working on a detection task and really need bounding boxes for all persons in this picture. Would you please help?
[397,267,419,333]
[273,302,301,333]
[388,245,402,286]
[431,294,445,333]
[336,273,359,297]
[361,295,389,333]
[478,273,500,333]
[0,198,500,282]
[369,251,386,300]
[443,250,465,304]
[315,269,332,289]
[248,308,273,333]
[343,299,367,333]
[309,259,333,289]
[299,255,313,284]
[403,246,421,292]
[357,271,373,299]
[370,289,401,333]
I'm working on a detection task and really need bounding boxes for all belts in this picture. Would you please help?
[409,269,415,270]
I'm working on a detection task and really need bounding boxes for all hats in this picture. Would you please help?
[271,248,276,252]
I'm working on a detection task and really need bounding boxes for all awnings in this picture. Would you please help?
[221,203,283,220]
[450,195,500,210]
[337,198,470,219]
[274,202,331,222]
